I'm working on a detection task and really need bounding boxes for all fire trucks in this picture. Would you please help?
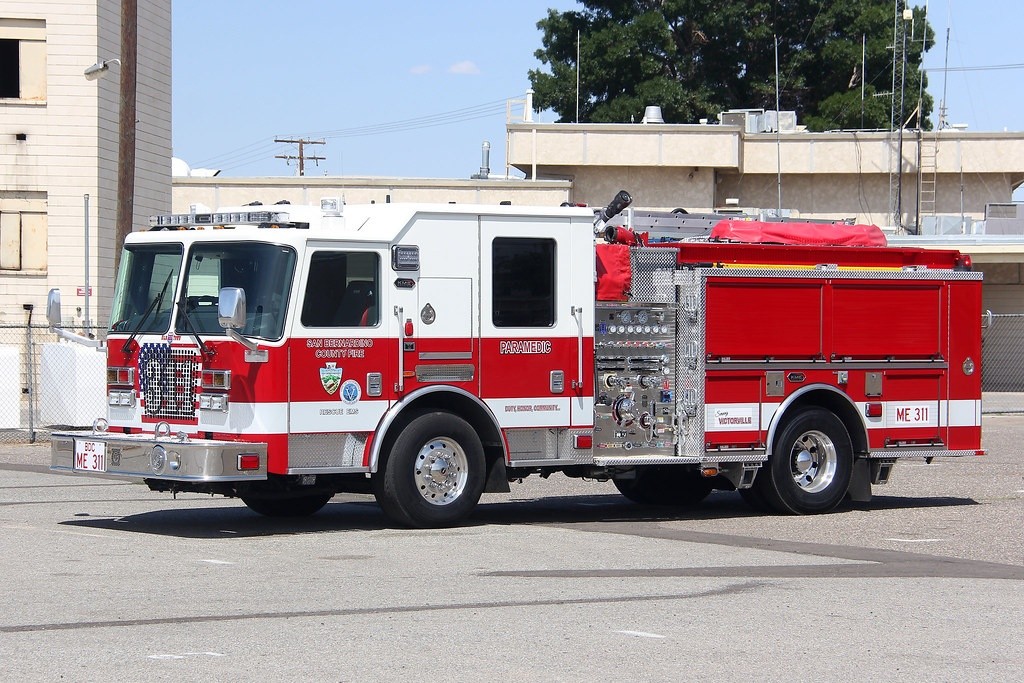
[44,186,989,530]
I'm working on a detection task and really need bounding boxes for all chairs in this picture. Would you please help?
[328,278,373,326]
[228,261,271,313]
